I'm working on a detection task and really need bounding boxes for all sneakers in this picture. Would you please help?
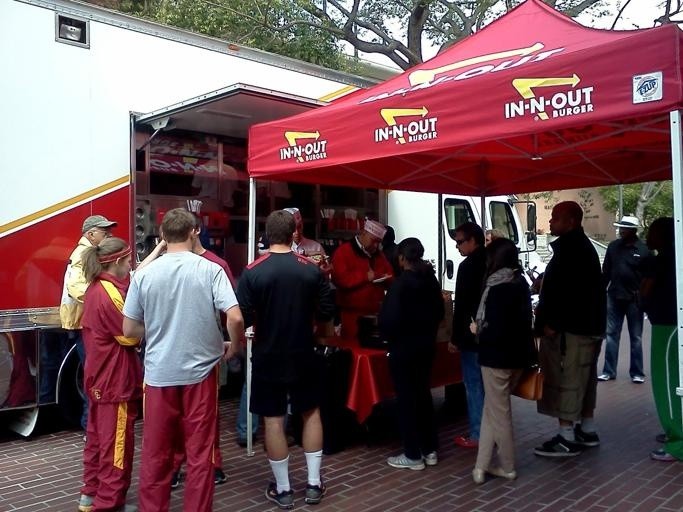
[655,433,666,442]
[420,450,438,466]
[532,433,583,457]
[78,493,95,511]
[631,374,644,383]
[649,446,678,460]
[171,471,181,489]
[597,374,610,381]
[387,453,426,471]
[573,423,600,446]
[214,468,327,511]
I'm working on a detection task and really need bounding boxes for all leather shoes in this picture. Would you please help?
[263,435,294,453]
[455,431,516,484]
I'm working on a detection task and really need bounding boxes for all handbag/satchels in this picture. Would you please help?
[511,366,545,400]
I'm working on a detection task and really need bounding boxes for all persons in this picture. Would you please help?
[16,222,78,326]
[376,237,446,470]
[234,210,334,509]
[600,215,655,384]
[135,211,233,488]
[121,208,245,512]
[192,141,238,258]
[636,217,683,461]
[447,222,535,484]
[60,216,118,443]
[532,200,606,458]
[78,236,143,512]
[235,207,398,452]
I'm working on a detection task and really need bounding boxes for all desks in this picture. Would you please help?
[324,336,468,450]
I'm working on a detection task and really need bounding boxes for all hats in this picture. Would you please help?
[81,215,117,233]
[612,215,642,228]
[282,207,302,225]
[363,219,387,240]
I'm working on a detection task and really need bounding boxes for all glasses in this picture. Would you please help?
[456,239,466,245]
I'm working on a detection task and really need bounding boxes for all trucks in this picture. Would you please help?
[0,1,538,442]
[527,243,553,285]
